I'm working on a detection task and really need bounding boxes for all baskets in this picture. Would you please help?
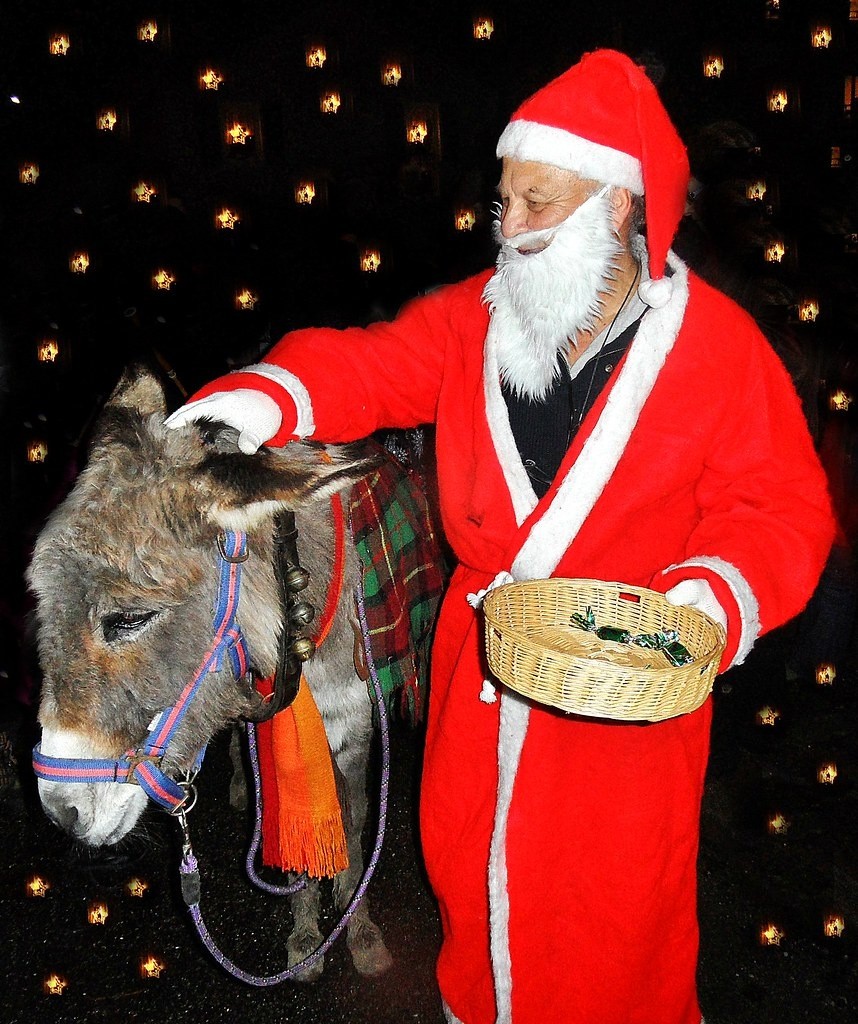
[480,577,727,724]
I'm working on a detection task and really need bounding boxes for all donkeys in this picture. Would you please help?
[26,364,448,987]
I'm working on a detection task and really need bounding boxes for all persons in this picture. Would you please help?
[162,52,834,1024]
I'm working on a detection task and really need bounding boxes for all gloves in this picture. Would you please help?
[664,573,732,641]
[161,386,283,456]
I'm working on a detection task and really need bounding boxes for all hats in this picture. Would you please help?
[494,48,691,308]
